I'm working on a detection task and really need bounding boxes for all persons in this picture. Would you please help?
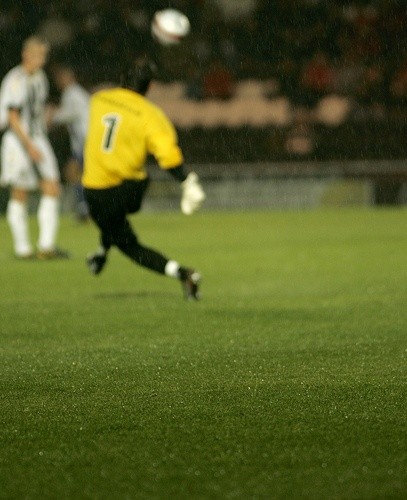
[0,33,68,259]
[47,57,91,223]
[82,58,206,301]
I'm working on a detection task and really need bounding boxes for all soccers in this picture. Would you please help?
[149,8,192,48]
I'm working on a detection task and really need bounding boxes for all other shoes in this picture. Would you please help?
[22,248,67,261]
[84,252,105,274]
[180,268,201,298]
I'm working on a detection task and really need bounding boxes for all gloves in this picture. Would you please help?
[180,172,206,216]
